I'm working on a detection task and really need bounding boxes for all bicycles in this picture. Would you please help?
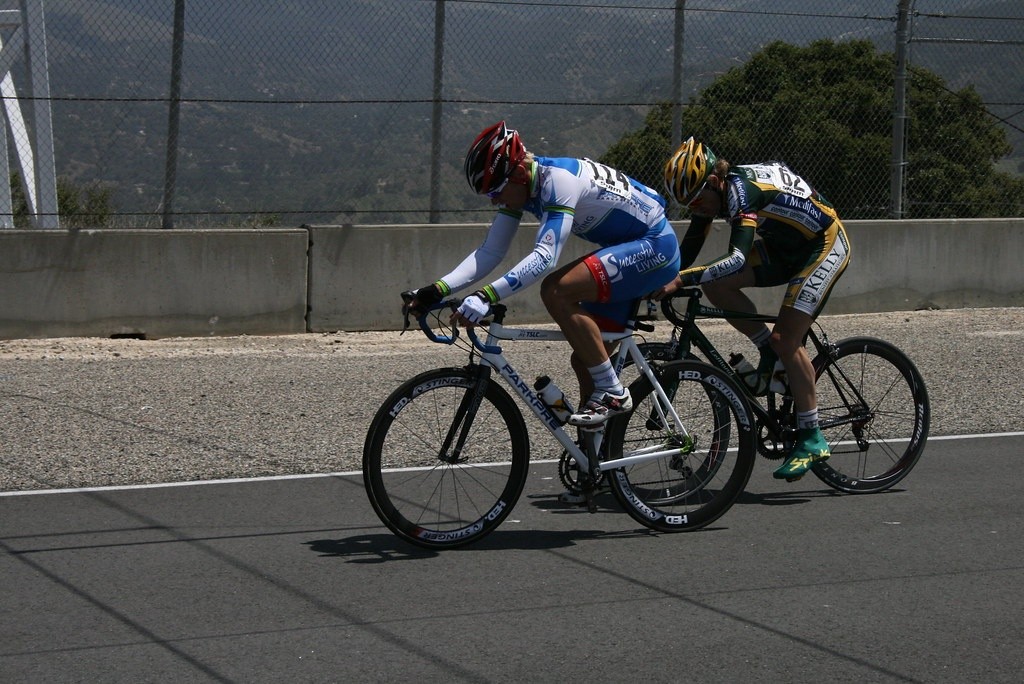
[359,271,761,551]
[605,280,931,507]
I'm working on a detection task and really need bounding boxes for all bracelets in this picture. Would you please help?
[482,286,497,304]
[436,281,449,297]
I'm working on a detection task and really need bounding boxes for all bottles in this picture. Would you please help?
[729,352,766,395]
[769,356,788,394]
[534,376,573,422]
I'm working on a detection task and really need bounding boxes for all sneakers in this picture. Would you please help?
[773,426,831,479]
[558,478,612,504]
[566,386,633,425]
[737,365,777,398]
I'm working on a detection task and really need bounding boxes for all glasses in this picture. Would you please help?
[485,177,509,198]
[687,180,709,208]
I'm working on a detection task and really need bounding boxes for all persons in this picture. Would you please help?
[400,121,681,505]
[652,136,851,479]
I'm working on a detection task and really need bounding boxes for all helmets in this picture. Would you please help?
[465,120,526,195]
[663,136,718,208]
